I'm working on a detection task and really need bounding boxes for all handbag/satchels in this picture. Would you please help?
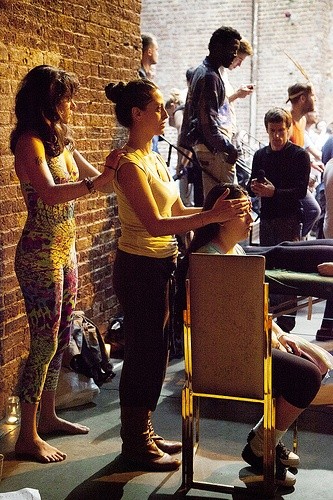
[54,311,124,410]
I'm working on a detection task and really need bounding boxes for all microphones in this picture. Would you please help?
[257,169,265,184]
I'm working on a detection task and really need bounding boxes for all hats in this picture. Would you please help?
[286,83,312,103]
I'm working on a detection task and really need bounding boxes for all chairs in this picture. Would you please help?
[182,253,298,499]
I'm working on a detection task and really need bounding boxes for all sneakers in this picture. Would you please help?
[242,429,300,485]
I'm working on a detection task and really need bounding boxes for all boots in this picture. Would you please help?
[120,407,182,470]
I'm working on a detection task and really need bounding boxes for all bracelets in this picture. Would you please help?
[82,177,98,194]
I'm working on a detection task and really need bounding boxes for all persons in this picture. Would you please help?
[164,27,333,341]
[104,79,251,470]
[140,34,162,158]
[178,182,328,485]
[10,66,131,463]
